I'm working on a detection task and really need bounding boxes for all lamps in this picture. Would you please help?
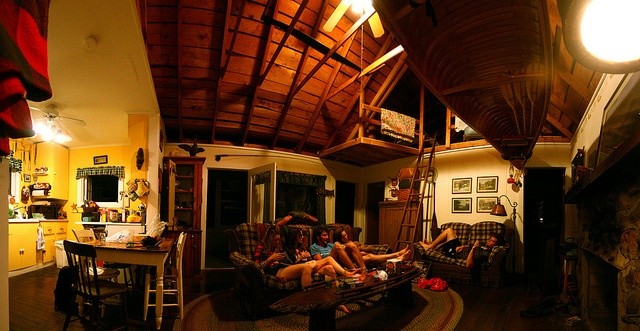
[490,195,520,283]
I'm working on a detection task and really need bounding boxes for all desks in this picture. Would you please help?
[269,265,422,331]
[580,247,640,331]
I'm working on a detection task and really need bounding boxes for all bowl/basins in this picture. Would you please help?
[131,233,145,242]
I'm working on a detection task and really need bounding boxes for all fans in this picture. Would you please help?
[322,0,384,38]
[30,104,86,138]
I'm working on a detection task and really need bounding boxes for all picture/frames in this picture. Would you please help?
[452,198,472,213]
[477,197,498,213]
[595,72,640,169]
[93,155,107,164]
[452,178,472,194]
[24,174,31,182]
[477,176,498,193]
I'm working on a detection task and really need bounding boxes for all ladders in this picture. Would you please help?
[394,134,438,252]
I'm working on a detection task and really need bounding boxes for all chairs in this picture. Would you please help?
[93,229,134,291]
[144,232,186,320]
[62,240,129,331]
[72,229,119,319]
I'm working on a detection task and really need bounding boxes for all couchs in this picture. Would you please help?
[415,221,502,288]
[225,222,391,322]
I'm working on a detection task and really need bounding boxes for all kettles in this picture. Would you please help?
[109,207,120,224]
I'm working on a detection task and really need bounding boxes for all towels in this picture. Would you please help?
[37,228,45,250]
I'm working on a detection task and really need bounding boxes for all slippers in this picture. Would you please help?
[418,276,442,288]
[430,278,448,291]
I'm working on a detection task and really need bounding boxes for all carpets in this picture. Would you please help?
[174,283,464,331]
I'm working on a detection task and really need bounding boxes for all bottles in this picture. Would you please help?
[125,209,130,217]
[102,207,106,222]
[122,207,125,222]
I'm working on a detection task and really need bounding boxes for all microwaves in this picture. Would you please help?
[27,202,60,221]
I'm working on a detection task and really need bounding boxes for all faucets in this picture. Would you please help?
[13,205,26,212]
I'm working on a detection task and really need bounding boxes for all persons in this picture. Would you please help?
[310,227,366,273]
[419,227,506,268]
[287,227,361,314]
[254,225,318,289]
[334,228,412,268]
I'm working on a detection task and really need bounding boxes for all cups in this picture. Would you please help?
[100,233,106,246]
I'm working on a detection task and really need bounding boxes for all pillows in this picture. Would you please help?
[230,252,264,276]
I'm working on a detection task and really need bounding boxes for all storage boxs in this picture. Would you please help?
[399,179,419,189]
[398,190,419,201]
[399,168,419,179]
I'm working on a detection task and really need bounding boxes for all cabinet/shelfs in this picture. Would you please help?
[40,222,67,266]
[164,156,206,273]
[377,201,422,244]
[8,223,37,278]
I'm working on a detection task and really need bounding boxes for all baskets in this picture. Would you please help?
[399,180,419,188]
[399,167,419,178]
[398,189,419,202]
